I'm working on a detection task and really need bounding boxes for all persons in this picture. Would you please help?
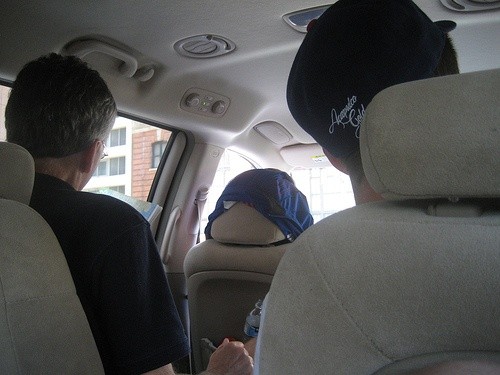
[4,52,255,375]
[285,0,459,208]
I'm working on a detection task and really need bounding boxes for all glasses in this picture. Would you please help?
[101,141,108,159]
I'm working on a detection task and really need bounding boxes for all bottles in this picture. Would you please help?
[242,299,263,344]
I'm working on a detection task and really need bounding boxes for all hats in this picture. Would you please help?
[286,0,461,159]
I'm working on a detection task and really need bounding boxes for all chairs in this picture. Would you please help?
[254,67,499,375]
[183,169,309,375]
[0,141,105,375]
